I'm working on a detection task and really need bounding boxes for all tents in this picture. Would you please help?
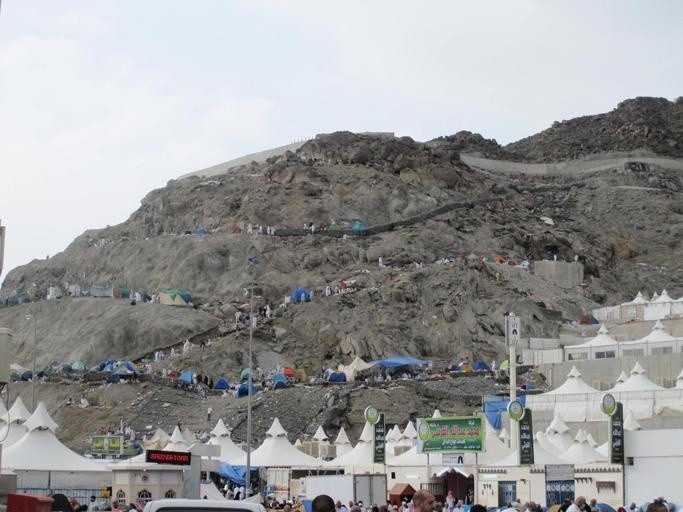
[0,335,525,394]
[580,314,597,323]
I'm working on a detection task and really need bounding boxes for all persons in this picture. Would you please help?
[53,469,682,511]
[373,249,583,273]
[0,277,378,335]
[82,218,362,250]
